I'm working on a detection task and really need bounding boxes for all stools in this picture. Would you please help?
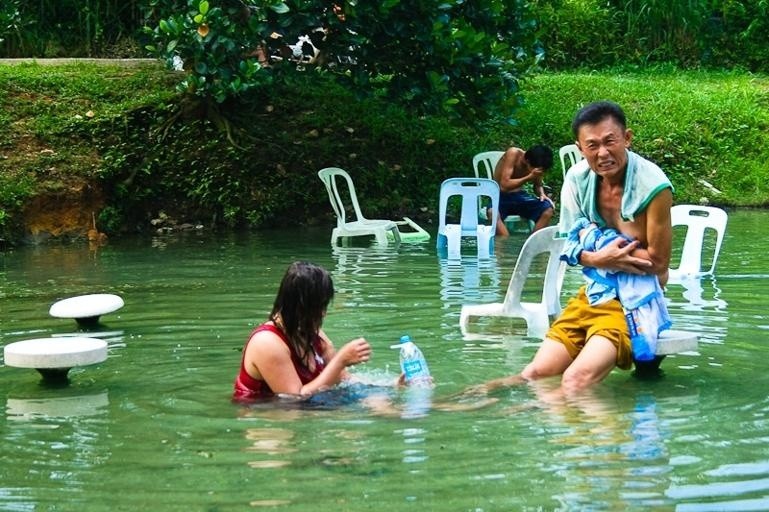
[49,293,125,326]
[631,329,697,373]
[3,338,108,380]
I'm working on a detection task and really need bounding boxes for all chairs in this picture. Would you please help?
[670,205,728,274]
[559,143,583,182]
[437,177,500,260]
[318,167,431,246]
[460,225,568,338]
[473,151,535,233]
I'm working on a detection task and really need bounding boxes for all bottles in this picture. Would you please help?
[399,335,431,389]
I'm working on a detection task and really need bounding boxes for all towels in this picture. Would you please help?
[553,148,674,240]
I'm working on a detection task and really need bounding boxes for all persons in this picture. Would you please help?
[472,103,672,417]
[233,262,502,419]
[486,145,556,237]
